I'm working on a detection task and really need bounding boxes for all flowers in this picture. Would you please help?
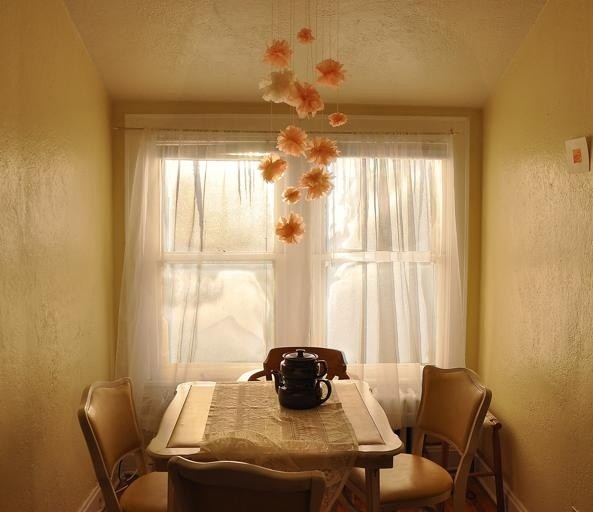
[256,1,347,244]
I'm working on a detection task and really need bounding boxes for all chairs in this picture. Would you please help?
[337,364,493,511]
[167,455,327,511]
[235,346,350,382]
[76,375,169,511]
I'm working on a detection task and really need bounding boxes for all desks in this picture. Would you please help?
[145,380,405,511]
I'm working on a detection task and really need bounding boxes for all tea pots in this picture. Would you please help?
[271,348,332,410]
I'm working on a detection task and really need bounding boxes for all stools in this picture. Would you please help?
[421,409,505,511]
